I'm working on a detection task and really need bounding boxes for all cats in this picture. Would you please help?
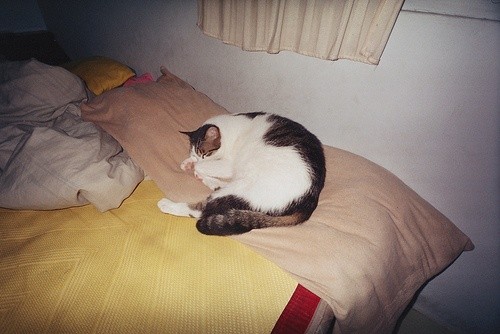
[157,110,327,236]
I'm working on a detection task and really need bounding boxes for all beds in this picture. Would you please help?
[1,29,335,334]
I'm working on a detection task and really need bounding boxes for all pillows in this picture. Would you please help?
[71,56,135,96]
